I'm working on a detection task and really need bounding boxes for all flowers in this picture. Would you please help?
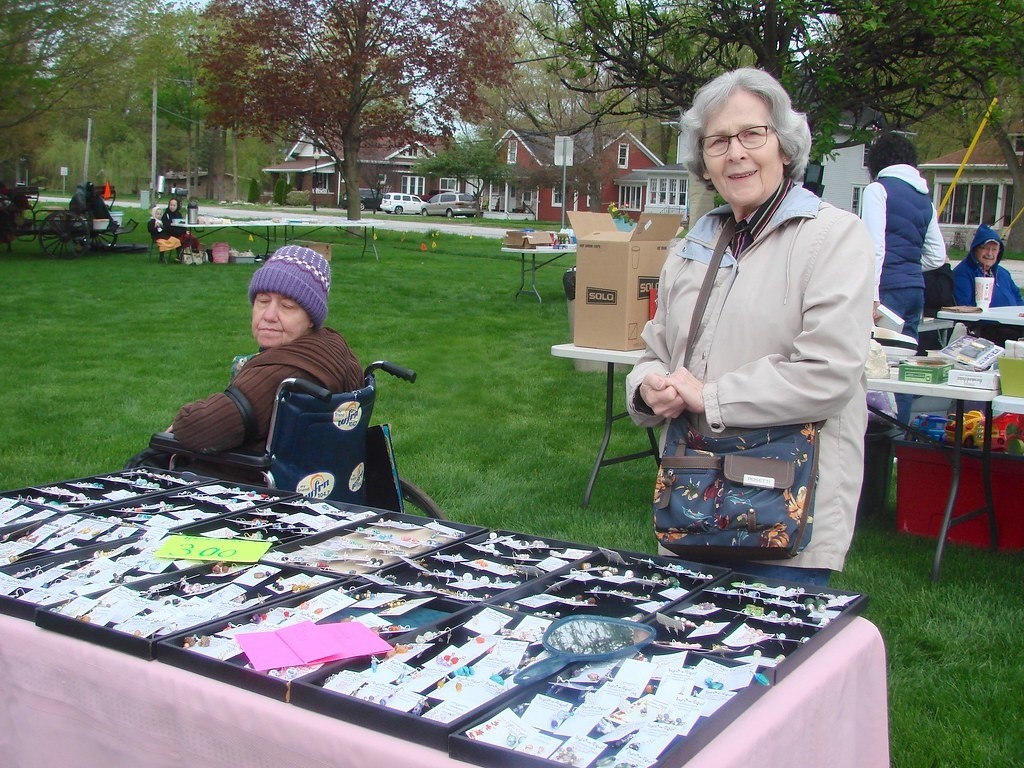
[607,201,634,226]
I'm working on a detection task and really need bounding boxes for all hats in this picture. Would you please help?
[249,244,331,328]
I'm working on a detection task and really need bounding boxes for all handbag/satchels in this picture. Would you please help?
[654,410,828,560]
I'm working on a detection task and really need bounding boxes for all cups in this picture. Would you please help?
[975,277,995,310]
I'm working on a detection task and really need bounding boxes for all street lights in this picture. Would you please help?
[312,153,320,211]
[20,156,30,187]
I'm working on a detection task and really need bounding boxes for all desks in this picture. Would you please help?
[170,221,385,261]
[501,247,577,304]
[0,615,891,767]
[552,305,1024,591]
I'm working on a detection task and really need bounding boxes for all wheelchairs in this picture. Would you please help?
[123,358,450,523]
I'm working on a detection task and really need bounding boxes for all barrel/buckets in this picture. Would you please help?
[109,211,124,227]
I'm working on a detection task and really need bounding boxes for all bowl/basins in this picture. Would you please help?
[172,219,179,223]
[93,219,110,230]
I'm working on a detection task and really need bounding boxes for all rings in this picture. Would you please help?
[665,372,670,376]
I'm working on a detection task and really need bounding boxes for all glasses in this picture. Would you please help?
[698,125,767,156]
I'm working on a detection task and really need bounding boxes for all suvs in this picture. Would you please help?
[339,188,384,211]
[421,192,481,217]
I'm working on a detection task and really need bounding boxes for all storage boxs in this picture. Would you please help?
[228,255,255,263]
[947,370,1000,390]
[999,356,1024,398]
[504,230,553,248]
[299,243,332,262]
[71,218,110,231]
[0,466,868,766]
[899,362,953,384]
[563,210,684,350]
[860,425,905,511]
[893,444,1024,552]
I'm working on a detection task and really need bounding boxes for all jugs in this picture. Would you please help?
[188,202,198,224]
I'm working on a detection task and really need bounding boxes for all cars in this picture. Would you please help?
[380,192,428,215]
[904,409,1024,453]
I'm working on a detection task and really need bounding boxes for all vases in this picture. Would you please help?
[612,219,636,231]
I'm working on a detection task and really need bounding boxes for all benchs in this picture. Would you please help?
[0,183,39,212]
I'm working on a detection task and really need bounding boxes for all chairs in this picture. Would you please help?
[147,222,169,264]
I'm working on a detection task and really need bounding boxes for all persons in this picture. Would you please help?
[916,222,1024,357]
[138,244,365,487]
[857,131,946,426]
[624,69,874,595]
[147,197,200,264]
